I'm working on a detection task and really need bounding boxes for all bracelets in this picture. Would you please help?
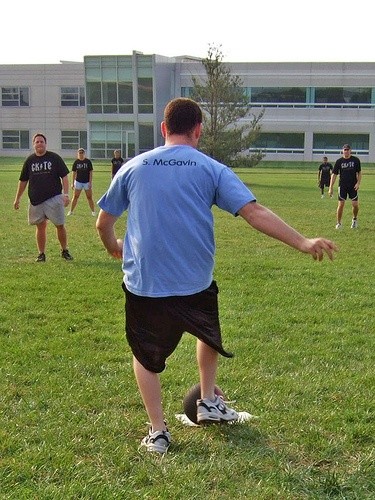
[63,193,70,197]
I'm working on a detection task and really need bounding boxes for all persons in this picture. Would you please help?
[318,157,334,199]
[329,144,361,229]
[110,150,124,179]
[94,99,339,453]
[14,133,74,262]
[67,148,94,216]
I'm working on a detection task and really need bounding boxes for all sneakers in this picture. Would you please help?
[196,394,239,424]
[141,425,172,453]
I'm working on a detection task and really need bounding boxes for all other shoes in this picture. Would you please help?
[351,219,357,228]
[36,253,45,262]
[67,211,74,216]
[321,195,324,199]
[92,212,96,216]
[335,224,341,229]
[61,249,72,260]
[330,196,333,199]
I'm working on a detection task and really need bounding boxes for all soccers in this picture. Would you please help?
[183,382,225,425]
